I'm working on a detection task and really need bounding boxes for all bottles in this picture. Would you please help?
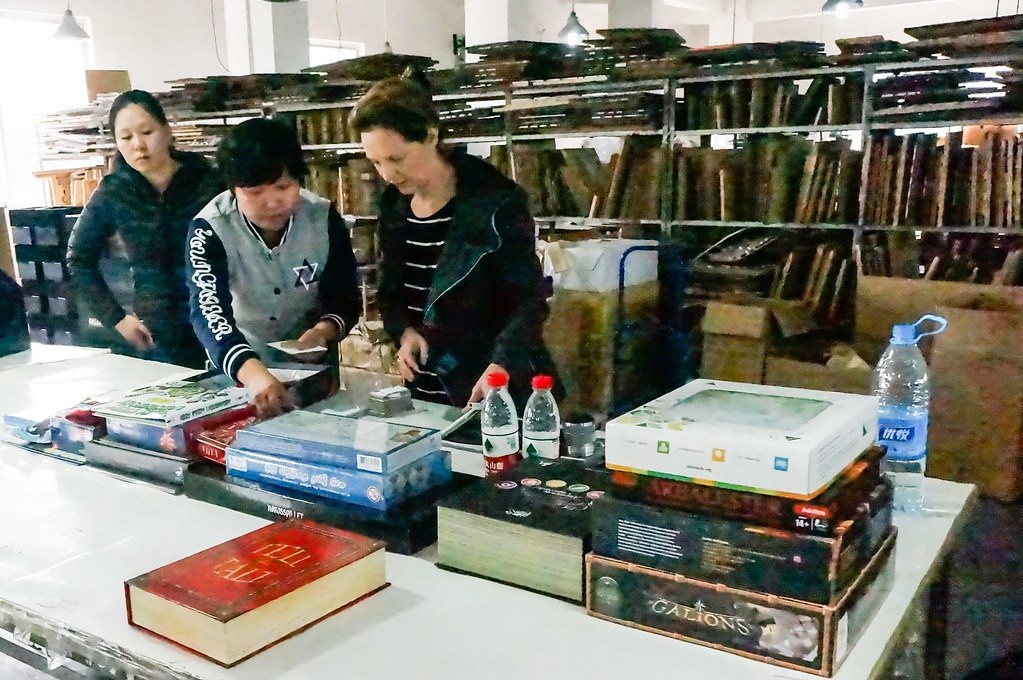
[522,374,559,461]
[481,372,519,482]
[869,314,948,513]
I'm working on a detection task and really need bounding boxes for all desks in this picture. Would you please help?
[0,349,980,680]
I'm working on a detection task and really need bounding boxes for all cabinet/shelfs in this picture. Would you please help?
[666,47,1023,274]
[271,78,668,238]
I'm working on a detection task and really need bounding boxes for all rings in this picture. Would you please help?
[394,356,401,362]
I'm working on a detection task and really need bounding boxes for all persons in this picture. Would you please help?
[67,90,220,374]
[183,117,363,419]
[347,78,568,413]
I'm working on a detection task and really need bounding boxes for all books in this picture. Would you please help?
[37,12,1023,331]
[1,359,489,556]
[125,515,393,670]
[434,377,899,679]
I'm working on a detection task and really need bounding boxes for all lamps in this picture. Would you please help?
[822,0,863,18]
[50,0,91,42]
[556,3,590,40]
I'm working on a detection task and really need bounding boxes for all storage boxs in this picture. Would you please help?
[584,524,898,679]
[760,333,888,395]
[544,281,661,414]
[105,362,336,459]
[928,290,1023,502]
[590,478,894,608]
[699,300,818,385]
[604,377,880,502]
